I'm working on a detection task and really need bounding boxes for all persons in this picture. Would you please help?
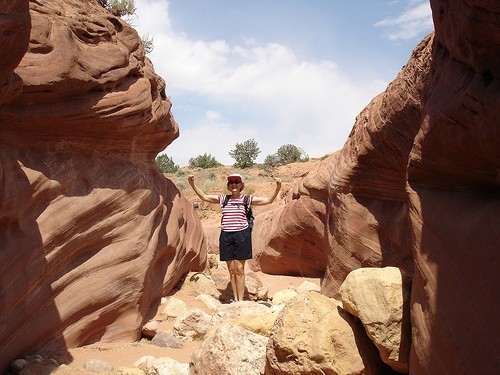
[188,174,282,301]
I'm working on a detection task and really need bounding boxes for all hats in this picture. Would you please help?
[227,174,242,180]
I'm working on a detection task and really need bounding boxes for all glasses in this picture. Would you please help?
[229,180,241,184]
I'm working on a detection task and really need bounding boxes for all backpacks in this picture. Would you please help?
[221,195,255,231]
